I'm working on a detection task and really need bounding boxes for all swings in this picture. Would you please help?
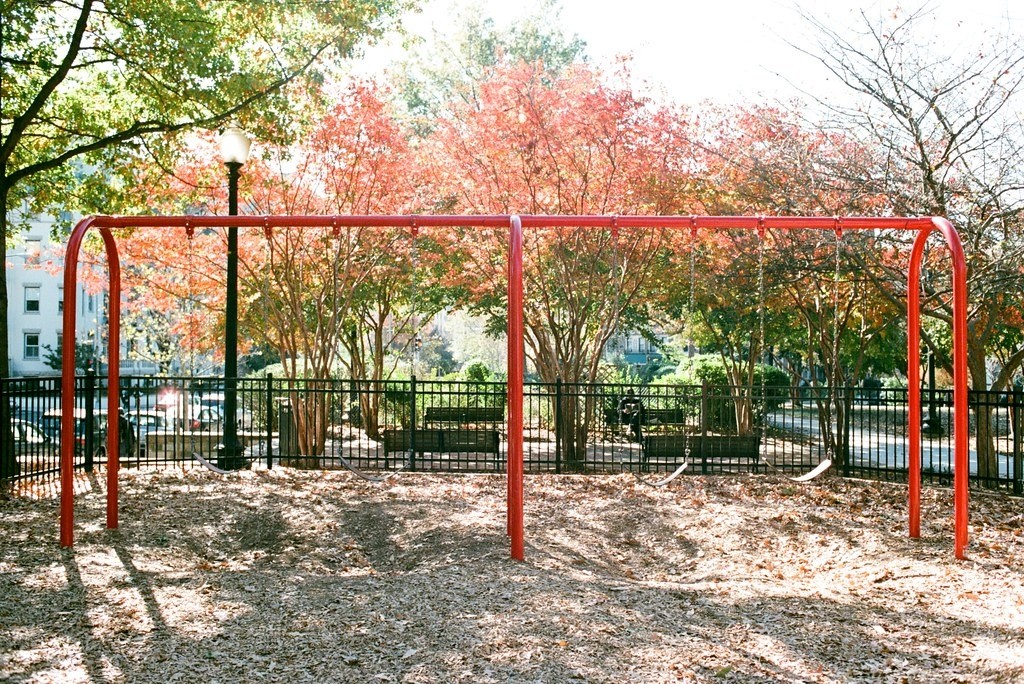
[752,240,841,484]
[332,239,417,480]
[611,238,697,488]
[185,234,276,476]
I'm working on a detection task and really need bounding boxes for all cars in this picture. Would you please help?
[10,391,255,459]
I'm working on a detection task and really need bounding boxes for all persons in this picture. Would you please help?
[617,388,645,443]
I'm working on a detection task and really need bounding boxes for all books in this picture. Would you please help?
[624,403,638,410]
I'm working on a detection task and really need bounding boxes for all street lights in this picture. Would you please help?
[216,121,255,470]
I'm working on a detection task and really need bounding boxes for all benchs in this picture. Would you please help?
[384,429,499,469]
[642,435,760,472]
[602,408,684,438]
[425,406,504,430]
